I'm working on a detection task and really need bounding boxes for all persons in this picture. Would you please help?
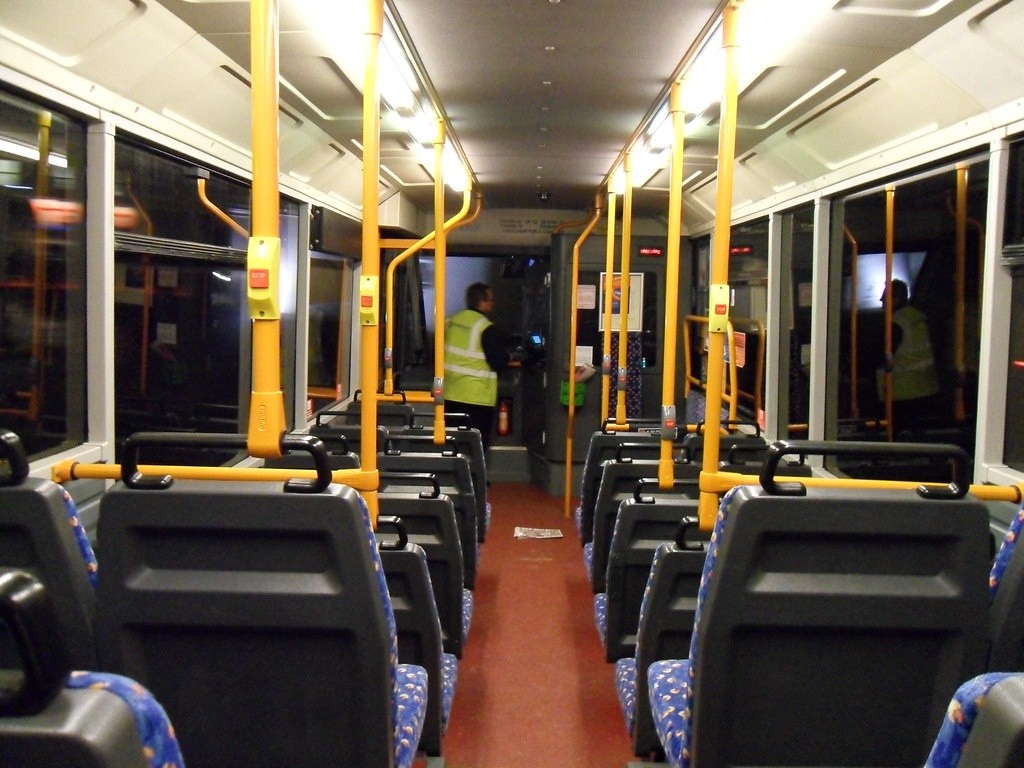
[876,279,939,436]
[442,282,516,487]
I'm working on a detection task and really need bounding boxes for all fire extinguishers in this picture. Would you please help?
[498,398,510,436]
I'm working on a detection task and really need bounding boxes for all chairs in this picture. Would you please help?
[576,416,1024,768]
[0,391,492,768]
